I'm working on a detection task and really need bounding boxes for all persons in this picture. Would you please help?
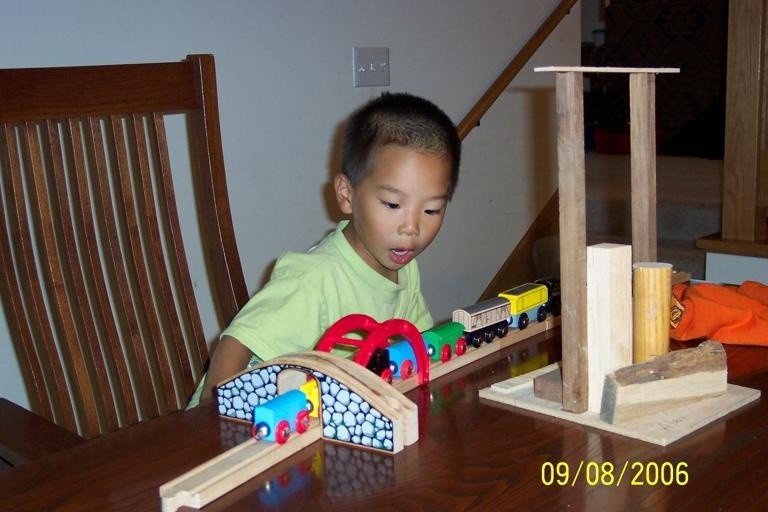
[186,90,460,411]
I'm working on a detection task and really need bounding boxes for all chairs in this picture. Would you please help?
[0,53,251,442]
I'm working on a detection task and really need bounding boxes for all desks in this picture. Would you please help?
[1,282,768,509]
